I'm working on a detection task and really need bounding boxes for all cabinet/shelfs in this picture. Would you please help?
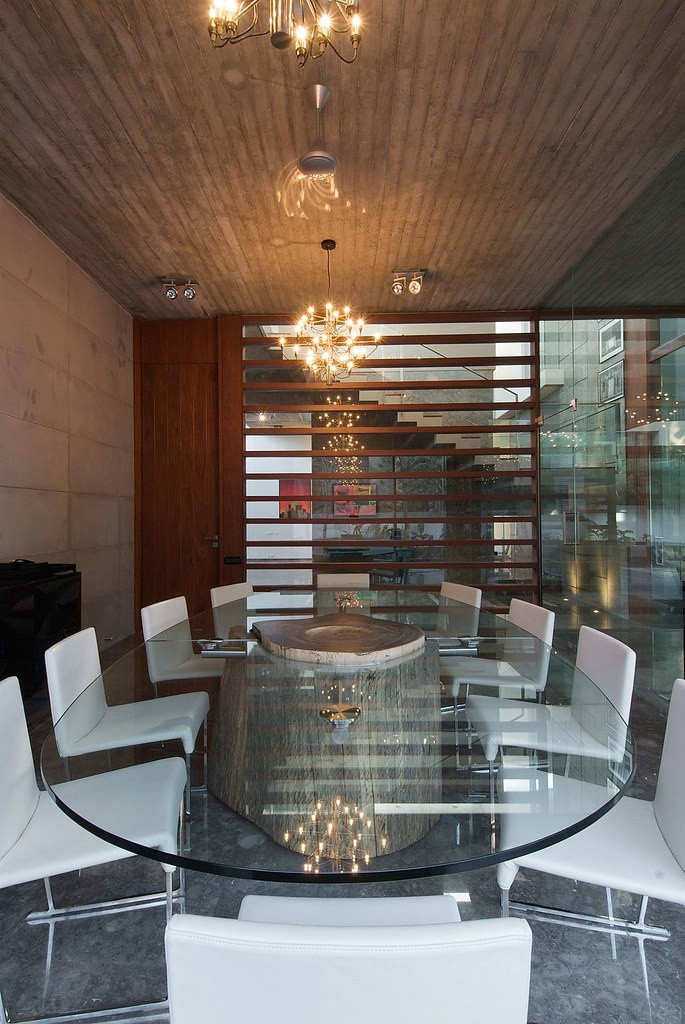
[0,560,82,697]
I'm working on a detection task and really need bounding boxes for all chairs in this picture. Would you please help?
[498,679,685,933]
[0,677,189,1024]
[210,582,254,641]
[45,626,210,851]
[424,581,482,711]
[440,598,555,771]
[165,896,533,1024]
[466,626,637,854]
[316,573,369,587]
[141,596,226,749]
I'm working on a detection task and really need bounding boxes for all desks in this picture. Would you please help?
[40,588,637,884]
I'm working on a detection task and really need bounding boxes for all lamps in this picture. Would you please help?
[209,0,362,68]
[408,272,425,294]
[391,271,407,296]
[162,279,178,299]
[184,280,199,299]
[278,240,381,385]
[276,84,366,221]
[318,395,364,486]
